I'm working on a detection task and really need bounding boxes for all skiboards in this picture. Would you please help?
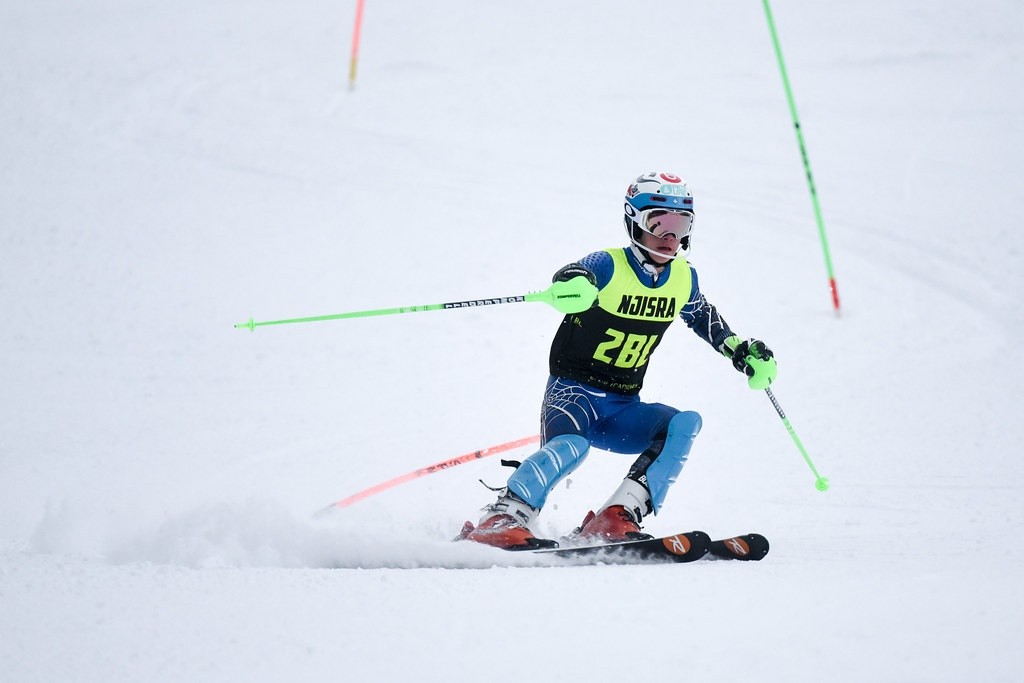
[452,528,770,562]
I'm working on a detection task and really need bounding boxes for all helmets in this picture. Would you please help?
[622,171,695,260]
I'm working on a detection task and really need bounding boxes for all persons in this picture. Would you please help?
[455,169,777,553]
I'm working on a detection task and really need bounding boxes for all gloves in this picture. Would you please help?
[723,335,775,378]
[551,263,601,309]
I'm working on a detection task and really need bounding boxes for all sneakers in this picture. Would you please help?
[571,505,654,541]
[449,515,560,550]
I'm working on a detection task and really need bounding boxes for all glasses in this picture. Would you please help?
[622,198,693,240]
